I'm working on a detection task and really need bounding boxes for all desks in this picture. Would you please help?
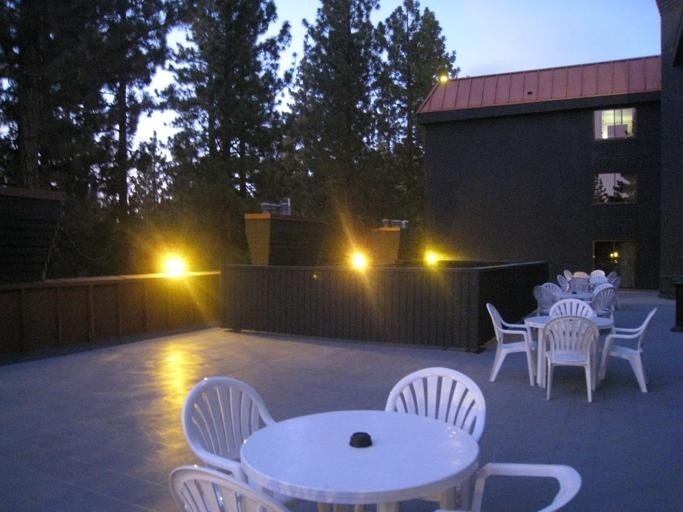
[240,410,480,508]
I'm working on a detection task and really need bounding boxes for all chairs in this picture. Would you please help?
[383,367,487,502]
[168,375,295,510]
[484,268,658,403]
[469,462,579,508]
